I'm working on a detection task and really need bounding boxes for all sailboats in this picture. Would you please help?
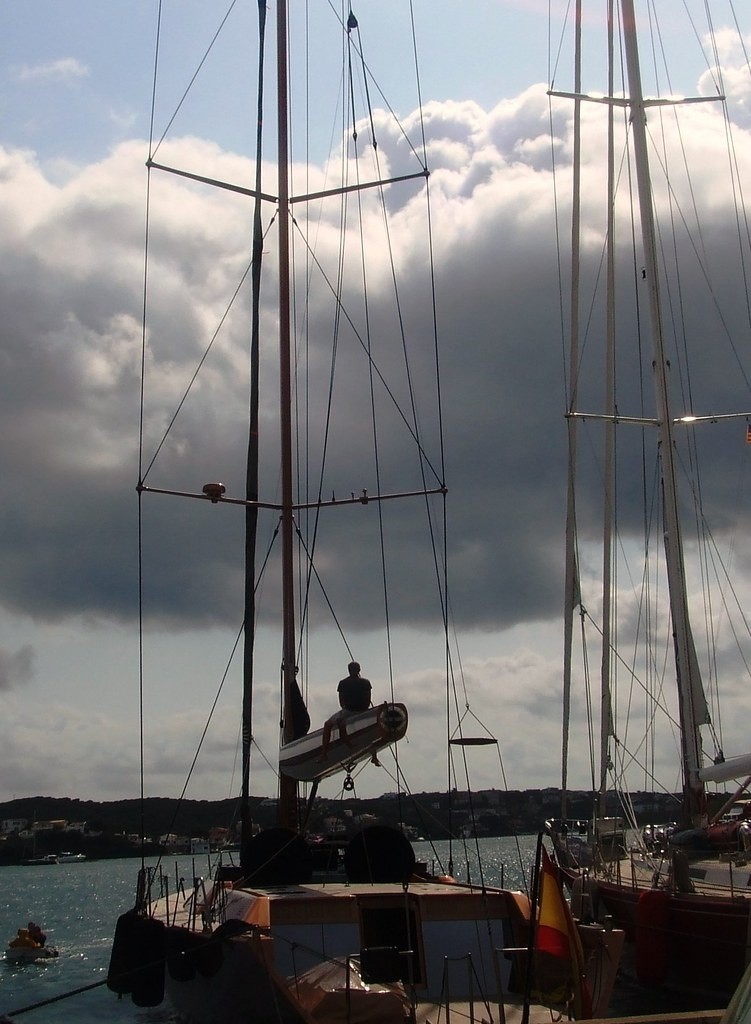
[101,0,751,1024]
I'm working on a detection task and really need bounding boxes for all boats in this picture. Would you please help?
[5,945,60,959]
[25,850,87,866]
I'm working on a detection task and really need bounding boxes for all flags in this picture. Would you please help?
[533,846,594,1021]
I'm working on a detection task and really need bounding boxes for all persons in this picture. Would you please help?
[9,922,47,949]
[314,662,381,767]
